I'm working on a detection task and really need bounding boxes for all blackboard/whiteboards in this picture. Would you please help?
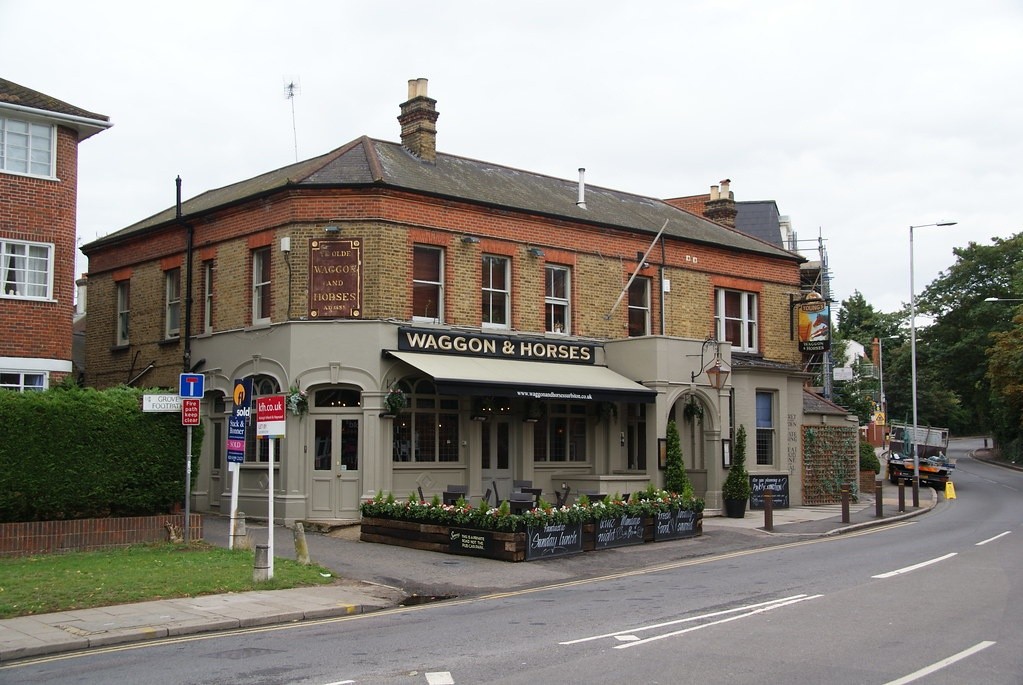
[595,512,645,551]
[527,519,582,562]
[751,475,789,508]
[654,508,696,542]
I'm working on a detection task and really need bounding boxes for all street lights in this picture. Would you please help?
[911,222,957,475]
[880,336,900,449]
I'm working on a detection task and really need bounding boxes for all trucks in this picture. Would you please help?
[887,419,950,486]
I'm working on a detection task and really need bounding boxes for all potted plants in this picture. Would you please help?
[721,424,752,518]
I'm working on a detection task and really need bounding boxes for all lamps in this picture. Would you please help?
[324,227,339,232]
[691,338,730,388]
[527,248,545,256]
[462,237,480,243]
[522,417,539,423]
[642,263,649,268]
[470,415,487,422]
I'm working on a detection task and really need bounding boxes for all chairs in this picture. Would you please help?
[492,479,542,514]
[549,487,571,508]
[588,494,607,504]
[443,484,467,506]
[621,493,631,502]
[484,488,492,504]
[418,486,424,501]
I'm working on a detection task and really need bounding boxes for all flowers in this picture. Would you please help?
[285,385,309,422]
[385,386,406,416]
